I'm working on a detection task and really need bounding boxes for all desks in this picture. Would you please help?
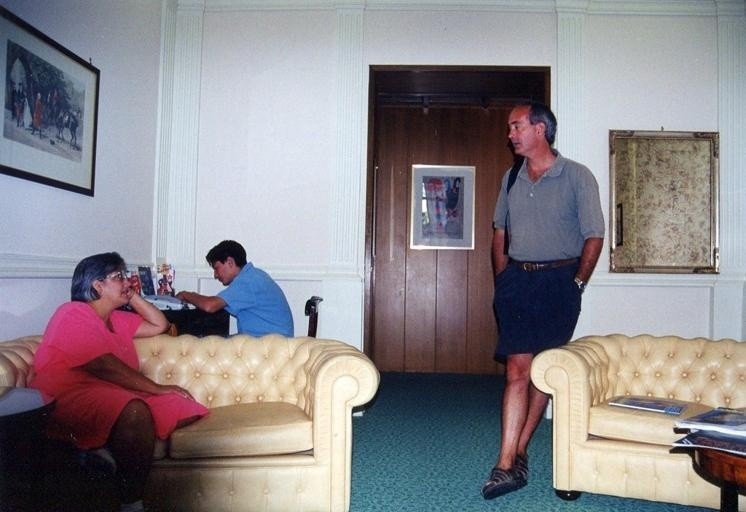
[120,307,230,337]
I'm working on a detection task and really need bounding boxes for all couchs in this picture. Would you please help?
[0,334,380,512]
[531,335,746,512]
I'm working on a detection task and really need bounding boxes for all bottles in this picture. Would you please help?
[129,272,140,297]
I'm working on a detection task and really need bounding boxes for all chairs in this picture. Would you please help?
[305,295,321,336]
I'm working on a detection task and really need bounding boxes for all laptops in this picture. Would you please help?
[135,266,196,308]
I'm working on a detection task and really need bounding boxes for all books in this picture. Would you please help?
[608,395,691,415]
[675,405,746,437]
[672,429,746,456]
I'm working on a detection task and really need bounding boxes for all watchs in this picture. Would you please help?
[572,277,588,292]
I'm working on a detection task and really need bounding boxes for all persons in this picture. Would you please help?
[23,250,212,511]
[176,239,295,338]
[9,77,80,153]
[479,100,607,499]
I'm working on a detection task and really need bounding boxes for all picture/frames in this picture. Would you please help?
[610,129,719,274]
[411,163,476,252]
[0,6,100,197]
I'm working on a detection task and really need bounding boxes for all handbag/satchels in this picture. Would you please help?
[492,211,509,253]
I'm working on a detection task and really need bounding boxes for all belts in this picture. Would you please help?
[508,257,580,273]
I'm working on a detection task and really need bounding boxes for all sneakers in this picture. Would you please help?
[482,467,521,499]
[514,452,529,484]
